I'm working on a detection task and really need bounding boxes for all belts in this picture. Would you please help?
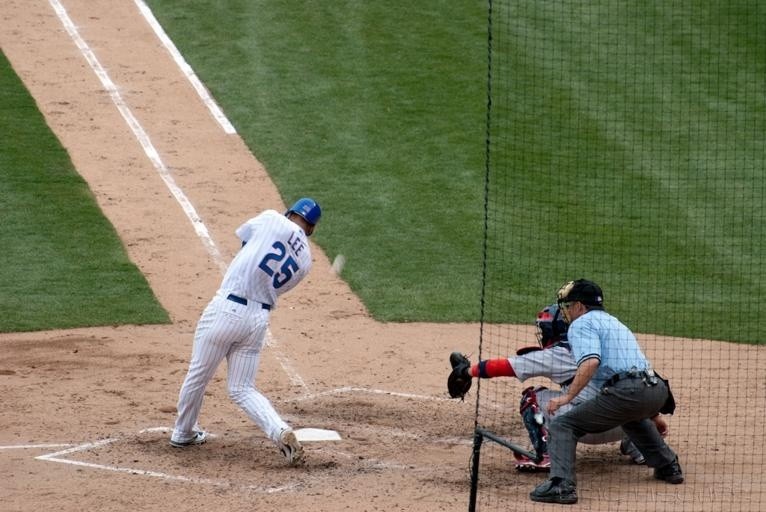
[601,371,655,390]
[226,294,270,313]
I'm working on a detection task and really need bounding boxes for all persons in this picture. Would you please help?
[529,278,683,505]
[165,196,323,467]
[442,304,668,474]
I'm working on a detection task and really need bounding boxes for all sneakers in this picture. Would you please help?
[511,452,552,472]
[278,427,305,467]
[653,462,684,484]
[530,480,577,504]
[619,442,646,464]
[169,430,208,447]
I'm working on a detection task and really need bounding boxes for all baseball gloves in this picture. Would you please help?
[447,352,472,398]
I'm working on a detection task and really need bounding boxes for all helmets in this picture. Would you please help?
[285,197,321,234]
[536,304,569,345]
[557,278,603,310]
